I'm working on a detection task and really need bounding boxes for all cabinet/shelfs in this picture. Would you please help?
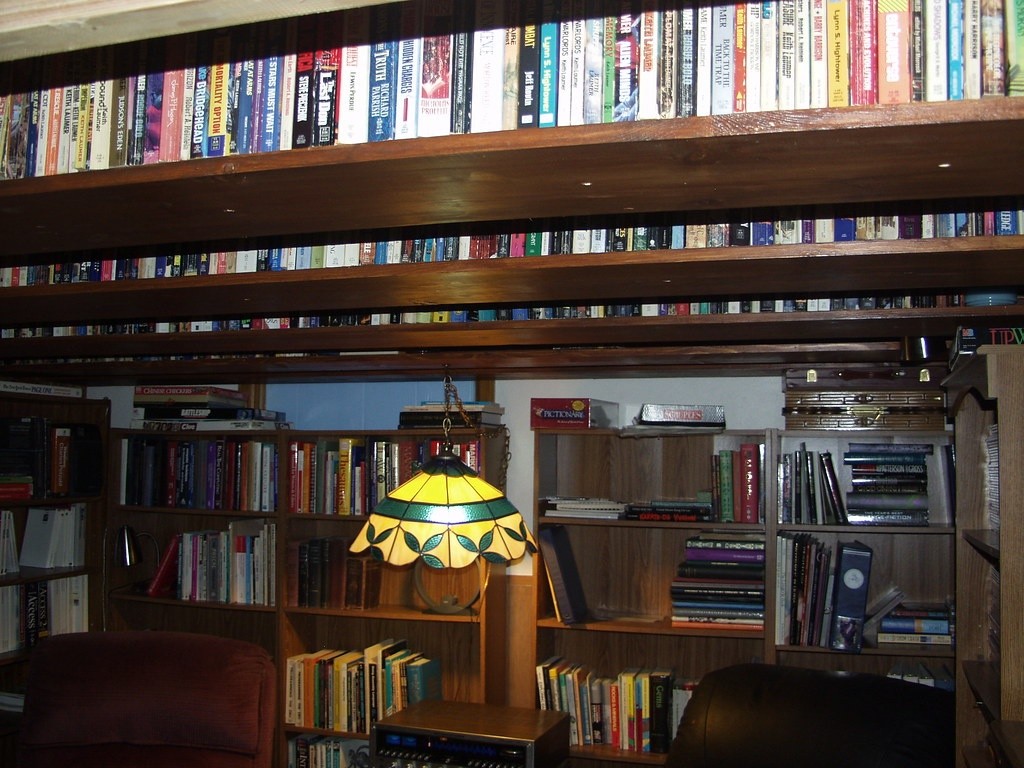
[0,95,1024,388]
[283,424,507,767]
[0,392,110,768]
[108,425,280,662]
[533,427,771,768]
[938,345,1024,767]
[771,429,958,692]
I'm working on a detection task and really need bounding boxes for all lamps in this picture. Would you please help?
[342,363,540,572]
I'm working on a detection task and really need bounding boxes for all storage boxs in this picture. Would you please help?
[531,397,618,431]
[639,403,726,427]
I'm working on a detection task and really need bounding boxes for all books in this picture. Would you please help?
[0,0,1024,371]
[1,375,1023,767]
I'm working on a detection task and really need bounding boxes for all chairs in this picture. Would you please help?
[662,663,953,768]
[14,630,277,767]
